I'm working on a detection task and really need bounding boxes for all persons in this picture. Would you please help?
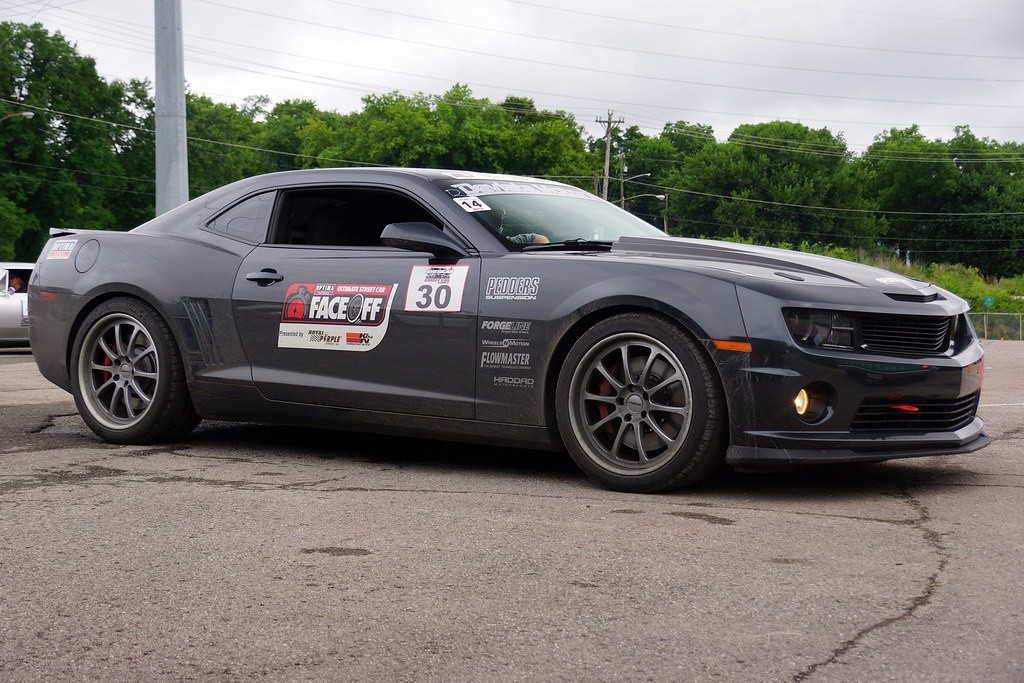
[8,271,27,293]
[477,195,551,246]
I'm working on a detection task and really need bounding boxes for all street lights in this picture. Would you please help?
[620,173,651,211]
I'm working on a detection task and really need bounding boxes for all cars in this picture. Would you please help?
[0,261,35,348]
[26,166,993,494]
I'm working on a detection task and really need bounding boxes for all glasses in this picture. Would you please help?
[9,274,19,280]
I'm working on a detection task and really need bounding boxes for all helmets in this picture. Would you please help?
[476,199,506,234]
